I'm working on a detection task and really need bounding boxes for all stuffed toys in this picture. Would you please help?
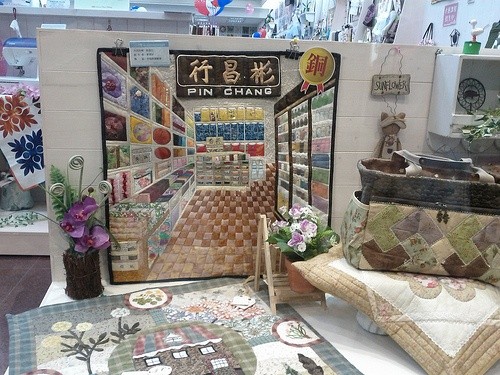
[373,112,406,158]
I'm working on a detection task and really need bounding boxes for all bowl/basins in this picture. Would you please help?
[463,41,481,55]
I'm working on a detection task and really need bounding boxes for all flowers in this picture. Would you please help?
[264,204,339,263]
[28,154,121,257]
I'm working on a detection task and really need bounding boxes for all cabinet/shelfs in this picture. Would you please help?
[427,53,500,139]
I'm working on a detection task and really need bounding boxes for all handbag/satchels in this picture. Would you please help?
[420,23,435,47]
[339,150,500,286]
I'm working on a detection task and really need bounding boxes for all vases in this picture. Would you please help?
[284,257,315,294]
[63,248,105,300]
